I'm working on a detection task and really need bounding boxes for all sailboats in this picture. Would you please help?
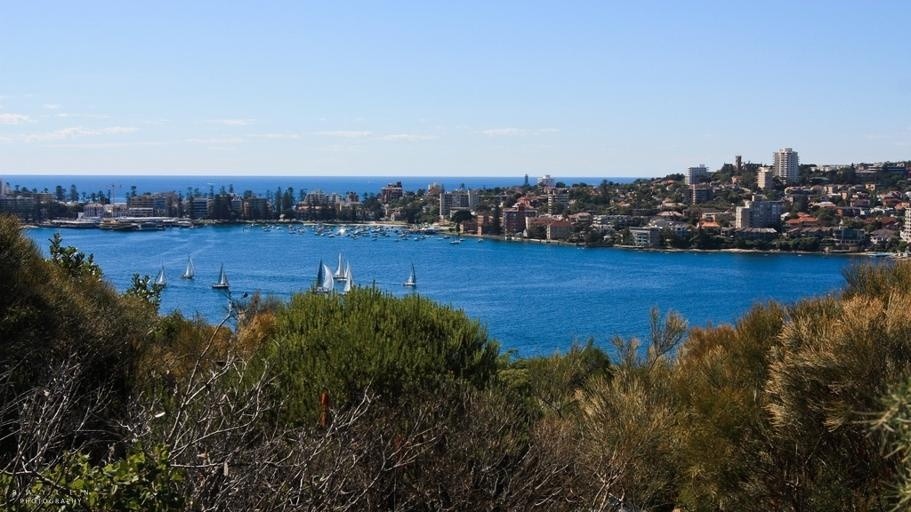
[402,261,418,287]
[150,264,168,289]
[212,262,233,290]
[182,255,197,279]
[309,252,359,299]
